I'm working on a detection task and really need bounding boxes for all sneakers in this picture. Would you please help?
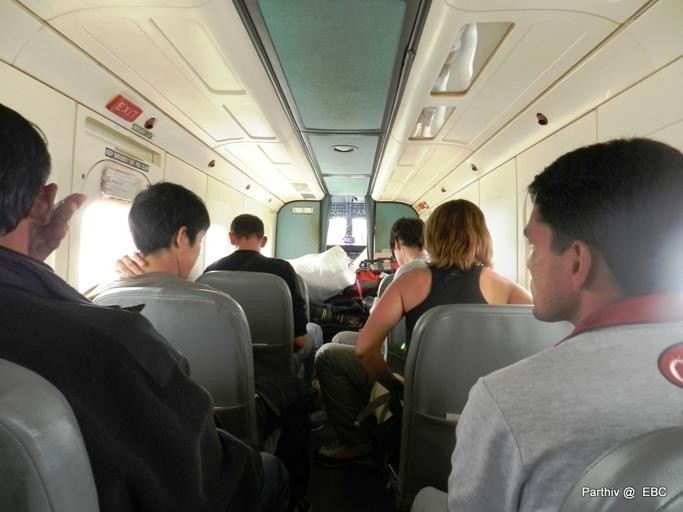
[315,438,372,470]
[307,408,328,430]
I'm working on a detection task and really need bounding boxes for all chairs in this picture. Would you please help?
[0,272,683,512]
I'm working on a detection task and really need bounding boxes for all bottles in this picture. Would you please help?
[336,314,364,329]
[320,305,328,324]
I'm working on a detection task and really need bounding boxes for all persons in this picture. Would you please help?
[332,330,361,346]
[104,183,328,448]
[0,101,291,511]
[355,198,533,424]
[313,215,429,471]
[202,213,324,432]
[411,138,682,512]
